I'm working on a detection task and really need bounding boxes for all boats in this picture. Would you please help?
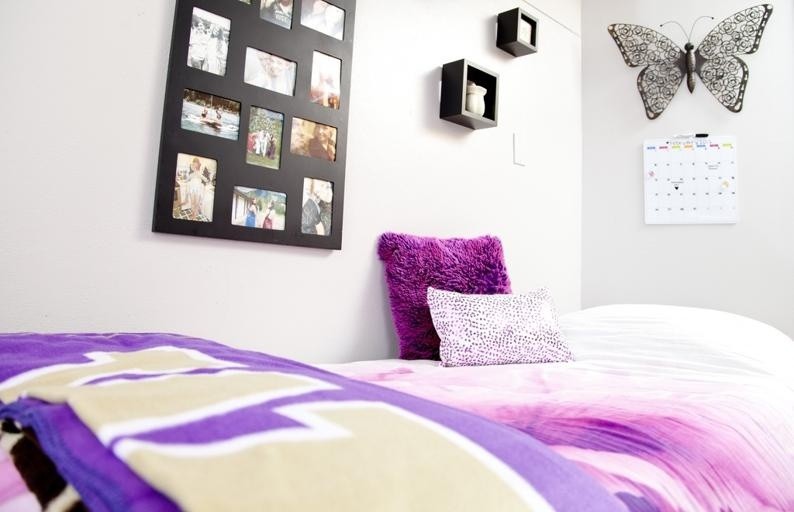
[202,117,222,126]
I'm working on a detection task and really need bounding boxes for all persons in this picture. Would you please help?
[244,197,276,229]
[187,19,226,75]
[201,109,222,122]
[246,129,278,159]
[307,123,337,161]
[305,1,343,38]
[248,50,288,93]
[270,1,292,25]
[302,192,331,234]
[185,157,211,220]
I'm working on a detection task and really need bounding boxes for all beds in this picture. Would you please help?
[0,307,794,512]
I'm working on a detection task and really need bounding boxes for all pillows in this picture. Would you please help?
[377,231,512,361]
[426,285,577,367]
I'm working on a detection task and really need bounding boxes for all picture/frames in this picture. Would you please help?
[151,1,356,251]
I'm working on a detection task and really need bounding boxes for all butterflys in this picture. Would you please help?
[606,3,774,121]
